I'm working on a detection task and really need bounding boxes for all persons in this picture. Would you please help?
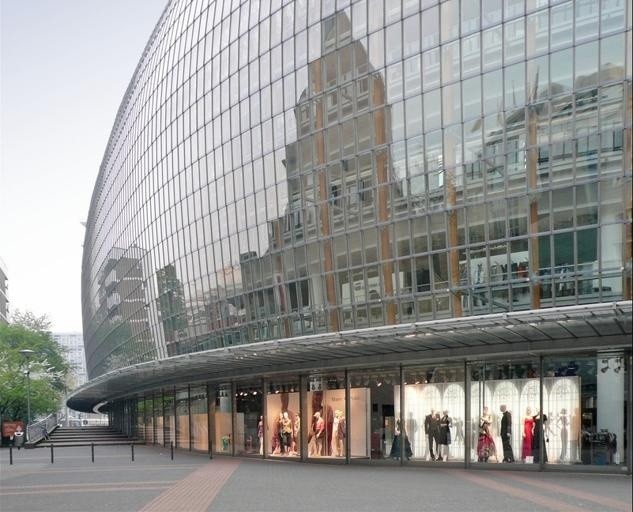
[499,404,516,463]
[258,415,264,454]
[520,406,550,463]
[425,408,453,462]
[270,394,299,454]
[333,410,347,457]
[307,391,333,456]
[476,405,497,463]
[387,416,413,461]
[470,263,487,307]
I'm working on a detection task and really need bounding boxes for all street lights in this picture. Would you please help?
[16,348,35,429]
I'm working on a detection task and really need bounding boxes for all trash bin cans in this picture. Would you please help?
[14,432,24,446]
